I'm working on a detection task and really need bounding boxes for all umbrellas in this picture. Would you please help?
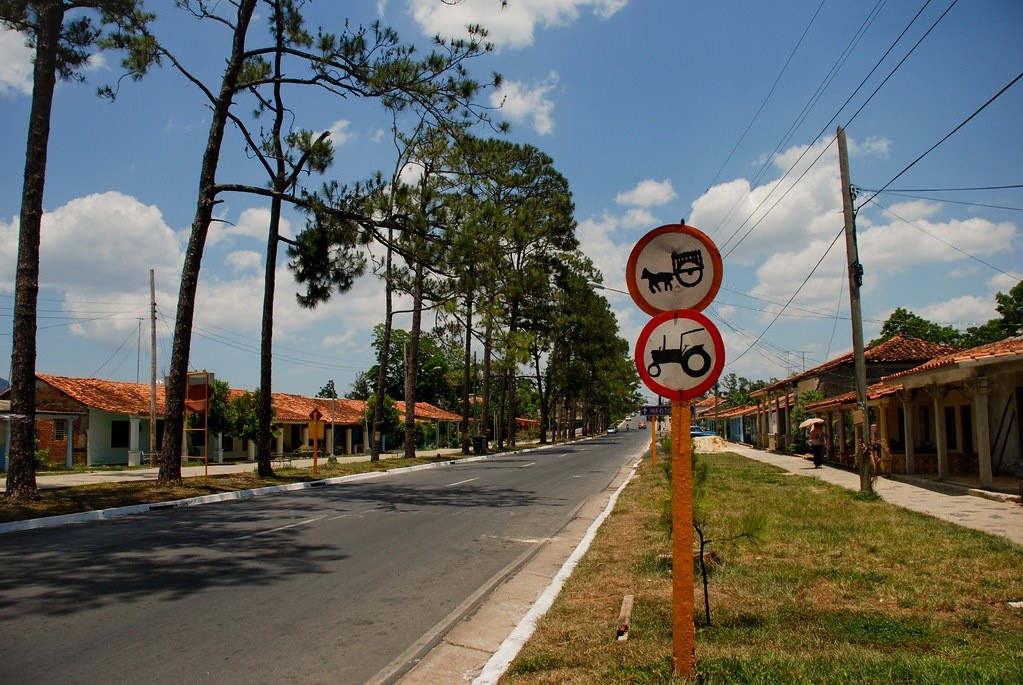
[799,418,824,428]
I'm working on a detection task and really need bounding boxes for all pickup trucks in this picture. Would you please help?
[691,426,717,438]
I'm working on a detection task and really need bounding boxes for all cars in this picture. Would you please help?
[625,411,641,421]
[607,426,619,434]
[638,421,646,429]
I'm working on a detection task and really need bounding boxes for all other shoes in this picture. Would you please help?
[817,466,822,469]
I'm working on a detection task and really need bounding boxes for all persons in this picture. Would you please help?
[810,423,824,469]
[745,424,750,442]
[626,423,629,431]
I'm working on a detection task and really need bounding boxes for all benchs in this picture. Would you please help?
[142,451,162,465]
[300,447,322,456]
[269,452,290,469]
